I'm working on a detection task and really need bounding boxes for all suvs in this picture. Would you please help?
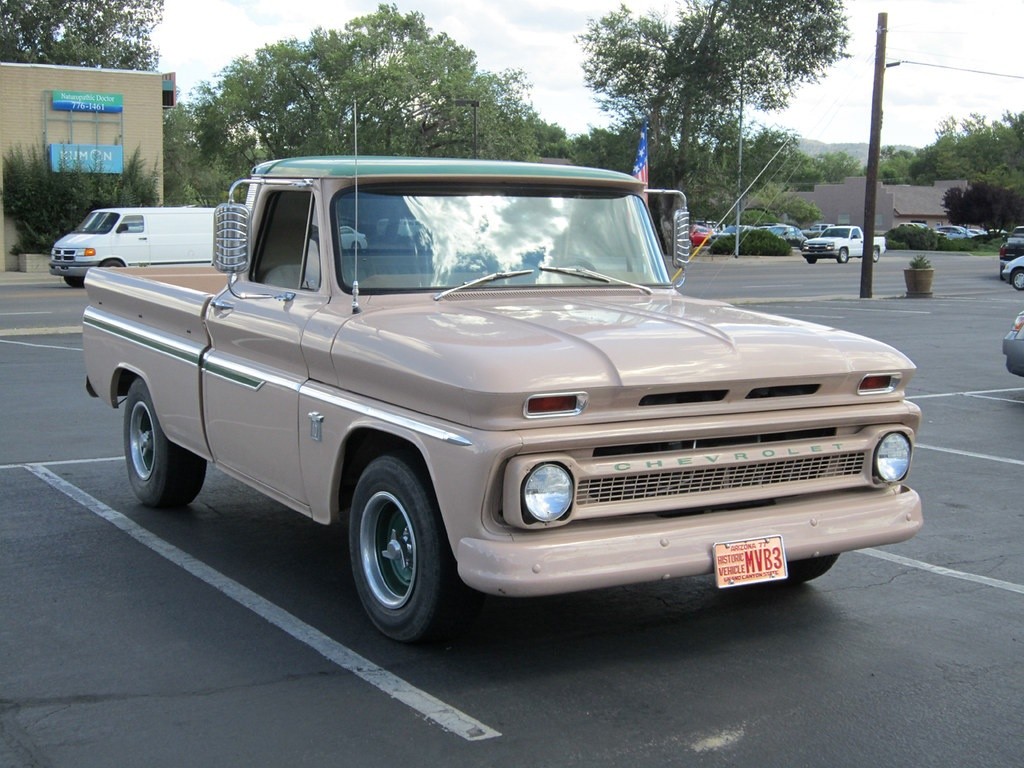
[936,226,978,240]
[897,223,947,239]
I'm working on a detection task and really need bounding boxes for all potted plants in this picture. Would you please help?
[903,254,934,292]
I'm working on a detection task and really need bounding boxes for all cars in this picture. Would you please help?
[340,225,368,250]
[689,222,834,251]
[999,226,1024,291]
[967,228,1008,235]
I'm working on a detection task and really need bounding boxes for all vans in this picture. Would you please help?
[48,206,216,289]
[376,218,423,242]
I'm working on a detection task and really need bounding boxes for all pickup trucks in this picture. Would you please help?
[82,154,926,645]
[801,225,886,264]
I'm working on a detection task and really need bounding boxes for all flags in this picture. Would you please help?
[633,117,648,205]
